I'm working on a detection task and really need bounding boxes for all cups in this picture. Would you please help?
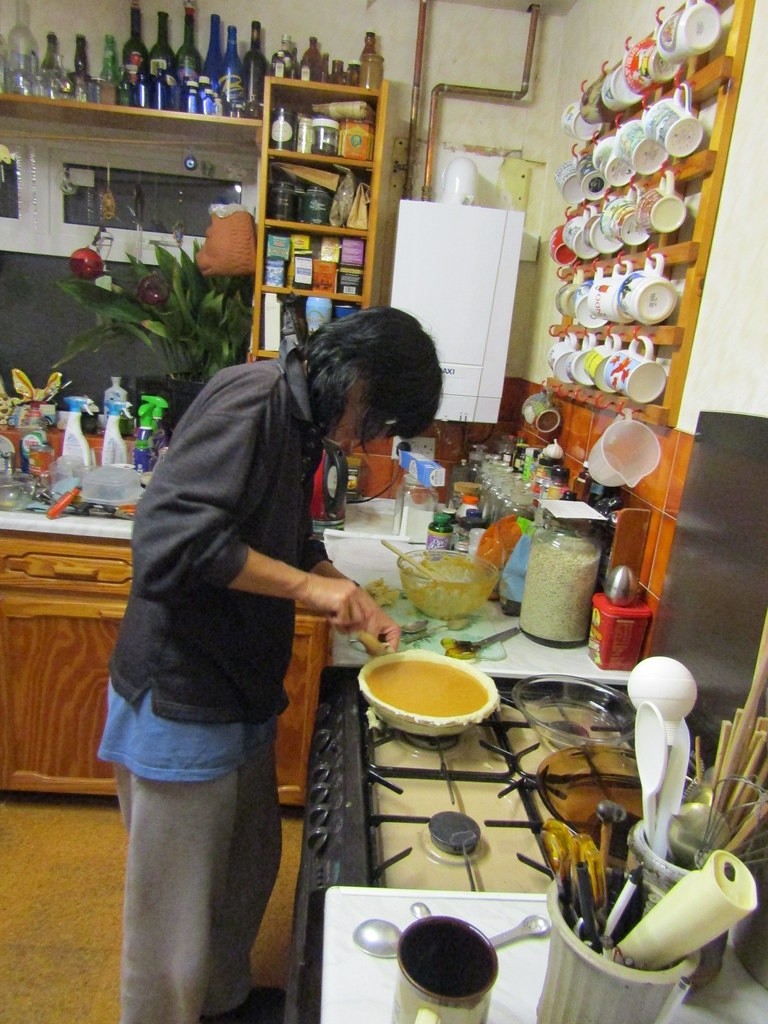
[518,0,721,434]
[390,915,499,1024]
[626,818,731,993]
[91,447,116,468]
[534,872,700,1024]
[38,455,84,504]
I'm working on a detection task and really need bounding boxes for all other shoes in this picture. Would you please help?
[200,985,287,1024]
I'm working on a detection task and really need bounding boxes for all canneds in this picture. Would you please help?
[28,444,55,479]
[269,107,339,225]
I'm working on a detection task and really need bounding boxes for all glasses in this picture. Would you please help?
[381,413,398,424]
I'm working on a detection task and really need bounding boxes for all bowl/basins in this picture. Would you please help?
[535,743,643,867]
[80,465,142,506]
[396,549,500,621]
[356,648,502,740]
[511,672,638,751]
[0,472,37,512]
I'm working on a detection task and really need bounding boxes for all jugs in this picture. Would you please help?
[587,407,662,488]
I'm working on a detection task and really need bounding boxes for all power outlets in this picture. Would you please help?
[391,435,436,462]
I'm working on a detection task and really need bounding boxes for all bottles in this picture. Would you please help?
[265,180,333,225]
[20,403,47,431]
[392,434,624,650]
[1,0,385,156]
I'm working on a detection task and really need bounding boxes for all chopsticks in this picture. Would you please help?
[692,611,768,859]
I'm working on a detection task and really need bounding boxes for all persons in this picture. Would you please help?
[96,307,442,1024]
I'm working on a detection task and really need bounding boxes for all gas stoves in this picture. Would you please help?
[290,666,647,985]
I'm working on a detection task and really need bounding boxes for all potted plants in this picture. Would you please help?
[57,234,248,432]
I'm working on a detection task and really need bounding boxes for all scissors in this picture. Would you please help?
[440,627,521,660]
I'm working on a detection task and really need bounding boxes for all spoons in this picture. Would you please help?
[487,916,548,947]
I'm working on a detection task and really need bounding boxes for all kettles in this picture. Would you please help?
[309,437,349,530]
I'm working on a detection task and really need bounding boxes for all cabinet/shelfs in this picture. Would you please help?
[0,529,143,797]
[249,78,390,361]
[264,593,327,813]
